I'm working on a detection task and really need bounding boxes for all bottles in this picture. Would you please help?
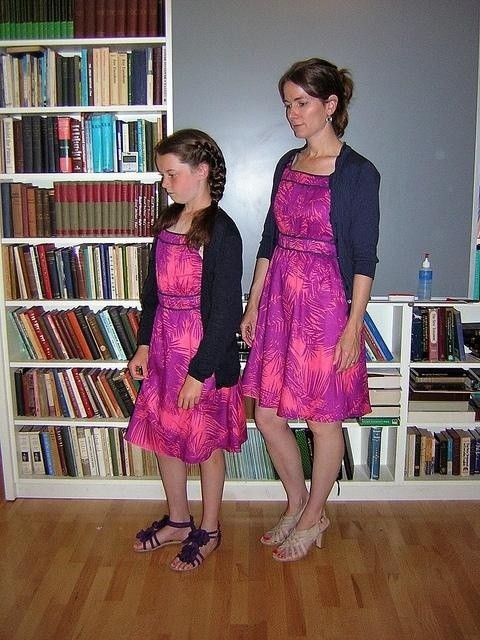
[418,252,433,299]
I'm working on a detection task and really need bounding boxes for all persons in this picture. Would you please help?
[239,60,380,562]
[123,131,247,570]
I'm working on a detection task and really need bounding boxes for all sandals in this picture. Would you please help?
[134,516,196,553]
[168,520,221,572]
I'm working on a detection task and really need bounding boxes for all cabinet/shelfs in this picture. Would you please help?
[401,301,480,499]
[184,300,410,500]
[0,0,173,502]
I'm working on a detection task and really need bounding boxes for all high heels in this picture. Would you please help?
[260,493,310,546]
[272,509,330,562]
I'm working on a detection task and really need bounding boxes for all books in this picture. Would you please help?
[1,112,167,174]
[15,425,161,477]
[1,46,167,108]
[223,428,281,480]
[336,427,383,480]
[407,368,479,423]
[362,309,394,363]
[5,306,142,361]
[2,177,168,238]
[388,294,415,301]
[410,306,479,362]
[14,367,142,419]
[186,463,201,476]
[291,429,314,480]
[1,0,166,41]
[237,302,255,423]
[404,426,480,476]
[356,368,402,426]
[2,243,152,300]
